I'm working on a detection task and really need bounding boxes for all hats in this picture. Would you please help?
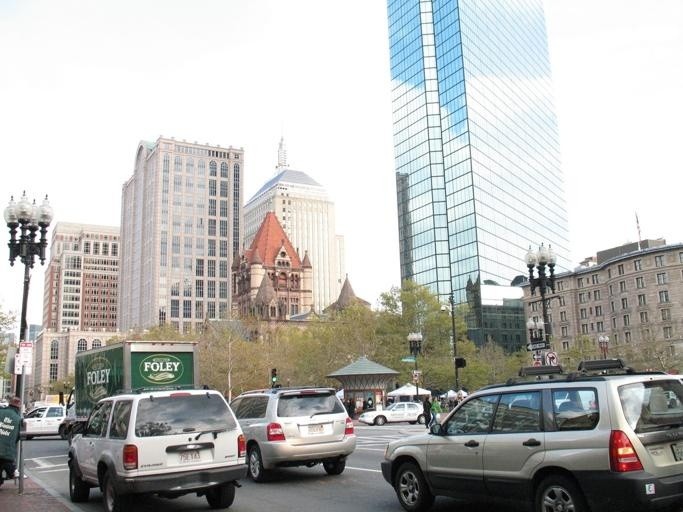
[9,397,21,409]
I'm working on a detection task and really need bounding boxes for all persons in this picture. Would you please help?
[343,398,356,418]
[423,398,431,428]
[440,398,465,412]
[0,396,24,485]
[430,396,441,427]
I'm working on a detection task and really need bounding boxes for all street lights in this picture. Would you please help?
[525,241,610,366]
[4,189,54,497]
[407,332,422,403]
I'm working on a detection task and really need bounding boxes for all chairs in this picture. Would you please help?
[559,402,578,411]
[511,399,530,409]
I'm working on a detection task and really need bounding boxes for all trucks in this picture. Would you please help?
[65,341,200,448]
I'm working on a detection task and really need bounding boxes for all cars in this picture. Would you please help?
[358,402,441,426]
[20,405,67,440]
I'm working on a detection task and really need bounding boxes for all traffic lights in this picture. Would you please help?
[272,368,277,383]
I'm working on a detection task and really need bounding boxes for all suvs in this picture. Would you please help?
[228,386,357,482]
[380,357,683,512]
[67,384,249,512]
